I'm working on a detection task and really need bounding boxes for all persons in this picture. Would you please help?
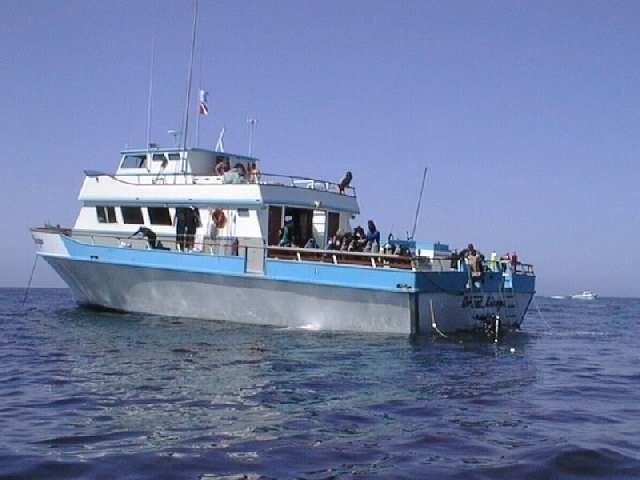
[216,157,225,177]
[249,162,258,185]
[279,216,517,275]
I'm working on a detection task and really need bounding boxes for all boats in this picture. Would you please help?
[551,289,599,302]
[30,129,536,337]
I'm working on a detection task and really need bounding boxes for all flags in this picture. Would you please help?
[199,89,208,115]
